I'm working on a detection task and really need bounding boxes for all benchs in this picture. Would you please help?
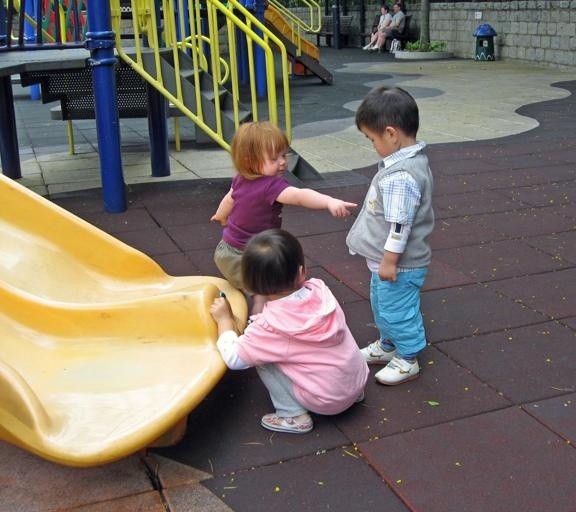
[306,15,412,54]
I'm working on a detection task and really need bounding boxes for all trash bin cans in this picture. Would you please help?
[472,23,497,61]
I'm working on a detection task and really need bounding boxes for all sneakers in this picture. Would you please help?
[375,356,420,386]
[261,412,313,433]
[362,44,378,51]
[360,339,397,365]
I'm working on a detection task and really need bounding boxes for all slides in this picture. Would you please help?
[263,5,319,62]
[0,174,249,468]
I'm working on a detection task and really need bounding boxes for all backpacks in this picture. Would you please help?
[389,39,402,54]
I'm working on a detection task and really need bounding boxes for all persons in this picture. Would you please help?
[345,85,436,386]
[362,5,394,55]
[208,228,372,436]
[368,2,408,53]
[208,119,362,318]
